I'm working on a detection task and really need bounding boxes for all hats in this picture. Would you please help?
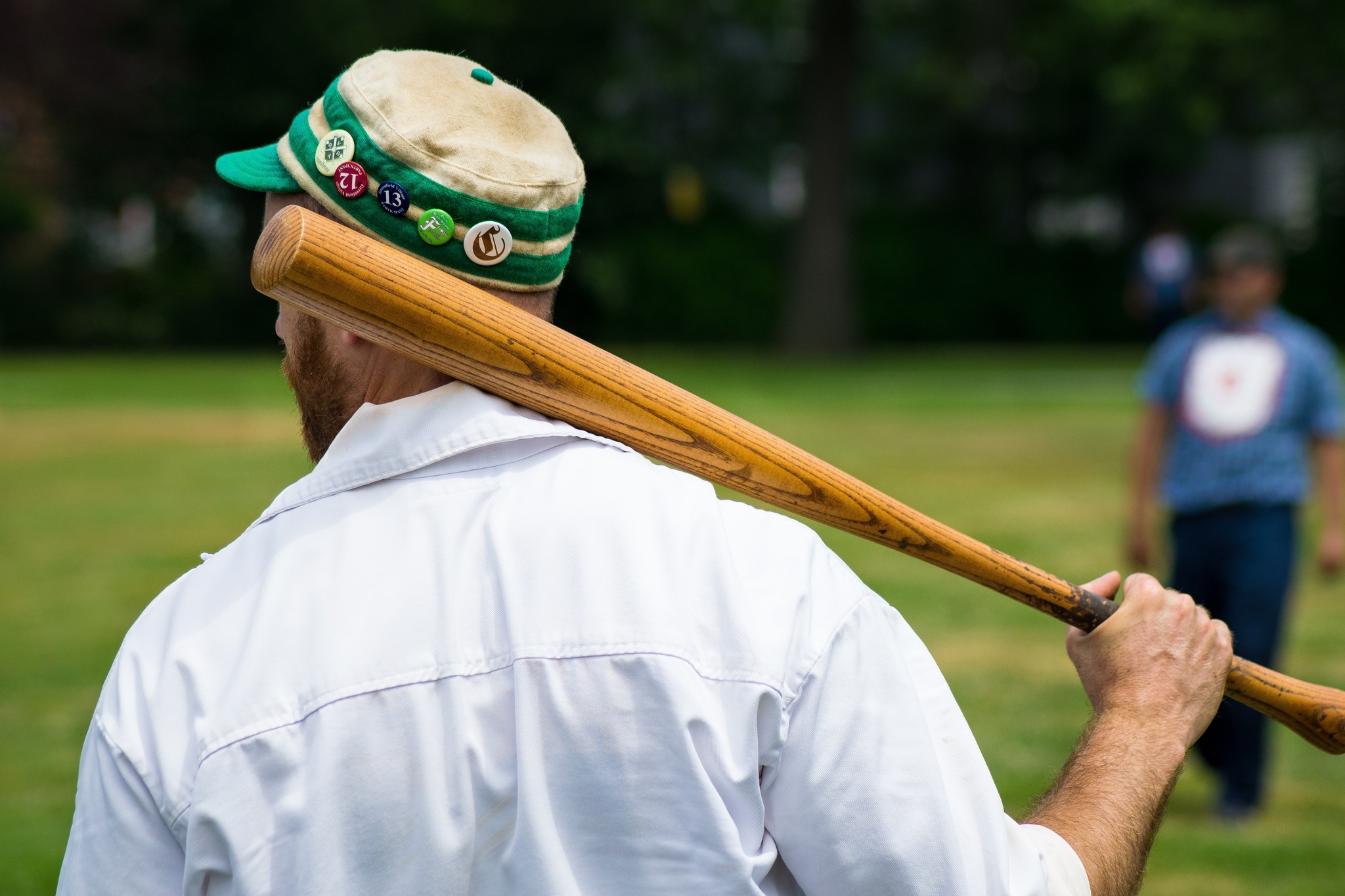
[1210,228,1285,272]
[215,50,586,292]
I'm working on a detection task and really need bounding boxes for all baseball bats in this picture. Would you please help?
[249,202,1345,755]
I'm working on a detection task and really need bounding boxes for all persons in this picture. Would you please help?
[1122,228,1345,818]
[54,47,1233,896]
